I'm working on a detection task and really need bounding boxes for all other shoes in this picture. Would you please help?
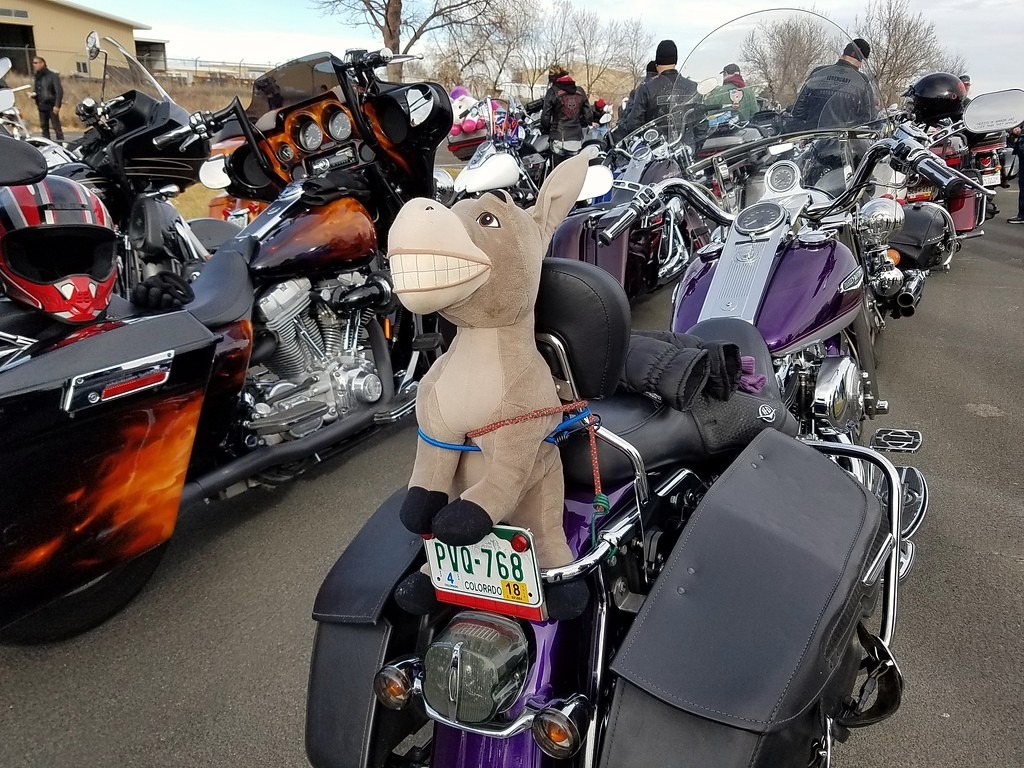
[1007,218,1024,224]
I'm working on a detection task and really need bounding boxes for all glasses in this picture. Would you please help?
[33,61,42,65]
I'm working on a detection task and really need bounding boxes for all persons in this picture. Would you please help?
[541,39,759,159]
[791,38,873,131]
[31,56,64,141]
[959,74,1024,224]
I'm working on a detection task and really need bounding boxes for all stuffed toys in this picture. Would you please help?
[385,153,590,620]
[451,87,486,135]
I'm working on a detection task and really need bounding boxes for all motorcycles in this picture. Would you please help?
[1,31,1024,643]
[302,43,930,768]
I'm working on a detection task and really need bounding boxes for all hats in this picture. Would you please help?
[845,40,870,63]
[596,98,605,108]
[720,64,739,74]
[656,40,677,65]
[646,61,659,78]
[959,75,970,84]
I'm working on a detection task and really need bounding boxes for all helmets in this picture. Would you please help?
[0,175,119,325]
[912,73,966,128]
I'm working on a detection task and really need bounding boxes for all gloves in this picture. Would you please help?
[616,339,710,412]
[134,270,196,311]
[633,326,742,401]
